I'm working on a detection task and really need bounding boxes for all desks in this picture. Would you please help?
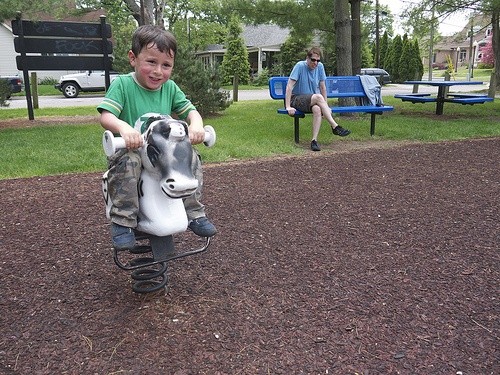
[404,79,484,116]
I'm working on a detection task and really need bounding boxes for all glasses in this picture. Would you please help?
[309,57,320,62]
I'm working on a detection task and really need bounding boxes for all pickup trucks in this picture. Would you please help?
[53,68,120,99]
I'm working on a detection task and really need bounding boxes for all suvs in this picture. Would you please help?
[360,68,391,85]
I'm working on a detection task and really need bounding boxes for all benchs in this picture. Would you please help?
[394,91,495,104]
[266,73,394,144]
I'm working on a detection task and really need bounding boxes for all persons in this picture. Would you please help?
[96,24,218,253]
[284,46,351,151]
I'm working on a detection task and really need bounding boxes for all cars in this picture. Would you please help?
[0,75,23,100]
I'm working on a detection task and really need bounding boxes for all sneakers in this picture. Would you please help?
[111,222,136,251]
[188,216,217,237]
[311,139,320,151]
[331,125,351,136]
